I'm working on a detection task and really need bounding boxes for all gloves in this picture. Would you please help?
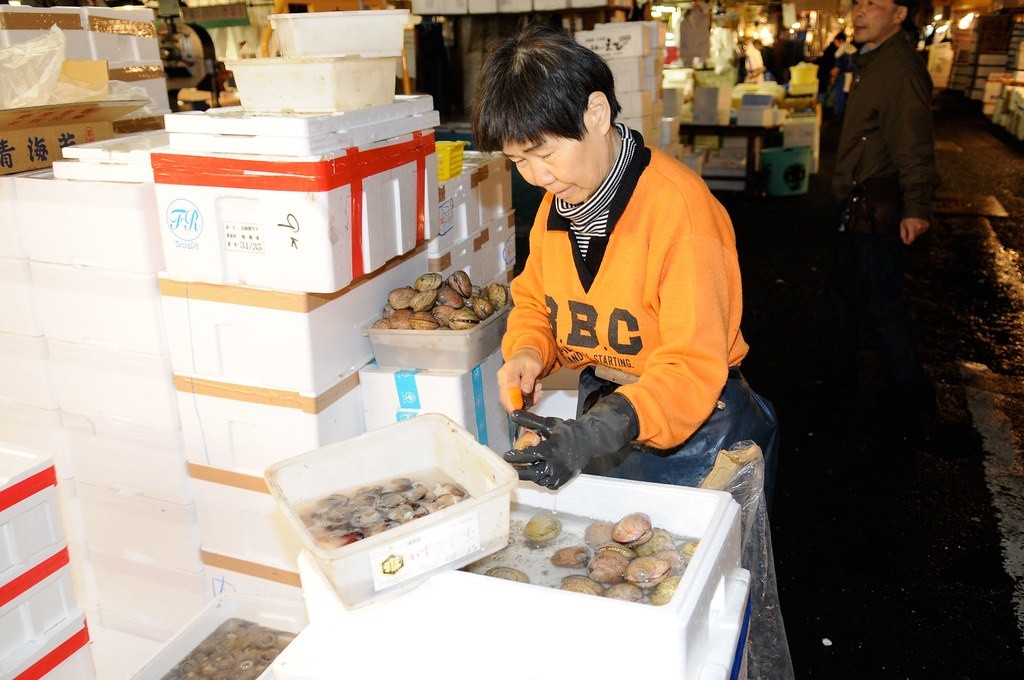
[504,392,639,490]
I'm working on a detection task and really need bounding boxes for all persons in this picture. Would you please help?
[793,0,939,401]
[472,25,781,621]
[709,10,949,126]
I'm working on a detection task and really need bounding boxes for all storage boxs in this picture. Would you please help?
[0,0,822,680]
[925,6,1024,142]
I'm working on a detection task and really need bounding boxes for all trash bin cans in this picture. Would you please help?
[760,144,812,196]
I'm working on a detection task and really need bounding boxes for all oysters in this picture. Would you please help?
[511,430,542,468]
[492,508,698,605]
[371,270,510,329]
[169,625,292,680]
[306,476,472,547]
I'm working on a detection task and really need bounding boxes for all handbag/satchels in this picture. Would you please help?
[839,178,903,234]
